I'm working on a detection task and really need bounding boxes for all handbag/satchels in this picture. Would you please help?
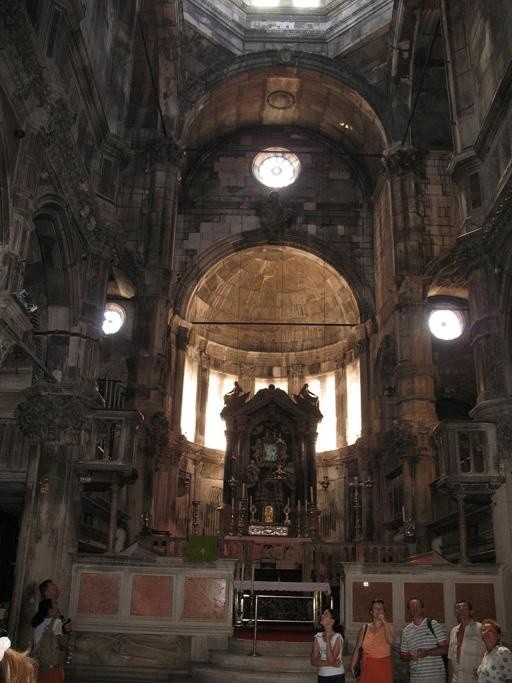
[352,645,364,676]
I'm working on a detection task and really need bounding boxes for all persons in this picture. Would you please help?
[311,608,345,683]
[351,597,512,683]
[30,579,72,683]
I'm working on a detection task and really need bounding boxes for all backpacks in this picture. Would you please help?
[35,614,61,672]
